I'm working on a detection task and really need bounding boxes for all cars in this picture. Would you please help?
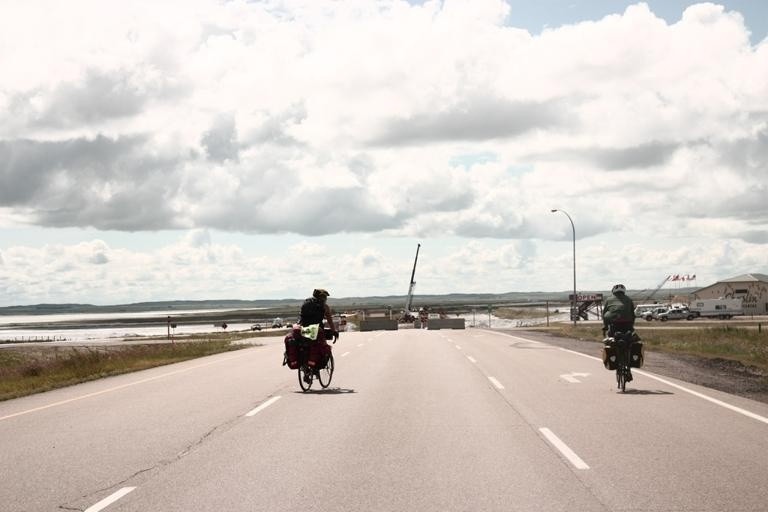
[252,324,261,331]
[272,322,294,328]
[643,307,697,321]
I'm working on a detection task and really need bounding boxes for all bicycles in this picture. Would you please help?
[299,329,336,392]
[602,327,633,392]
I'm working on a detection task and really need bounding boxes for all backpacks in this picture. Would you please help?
[298,299,324,327]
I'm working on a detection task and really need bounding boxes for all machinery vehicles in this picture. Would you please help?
[399,244,428,323]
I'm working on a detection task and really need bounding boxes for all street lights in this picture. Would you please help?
[168,316,170,340]
[388,305,392,320]
[551,209,576,327]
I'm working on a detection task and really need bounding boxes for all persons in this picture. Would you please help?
[300,289,335,384]
[602,284,635,380]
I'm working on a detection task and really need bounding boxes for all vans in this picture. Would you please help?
[324,316,347,332]
[634,304,664,318]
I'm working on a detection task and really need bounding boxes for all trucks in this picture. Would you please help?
[689,299,744,320]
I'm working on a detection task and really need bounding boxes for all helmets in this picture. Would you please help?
[612,284,626,295]
[313,289,329,298]
